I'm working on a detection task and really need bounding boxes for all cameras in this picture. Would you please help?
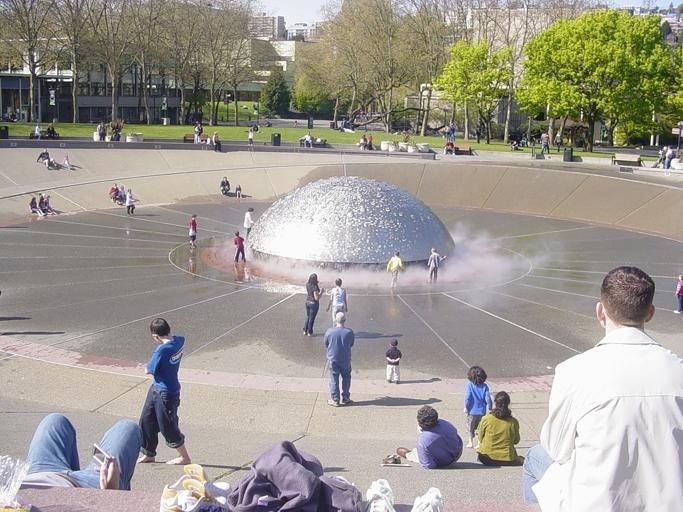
[91,444,117,471]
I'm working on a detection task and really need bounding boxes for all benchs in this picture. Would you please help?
[443,146,472,154]
[357,143,378,150]
[29,130,59,139]
[299,137,325,148]
[611,152,641,167]
[183,133,212,143]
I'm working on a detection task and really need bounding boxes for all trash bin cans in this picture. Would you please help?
[271,132,280,146]
[564,148,572,162]
[0,126,8,139]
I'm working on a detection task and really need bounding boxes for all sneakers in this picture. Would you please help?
[412,486,444,511]
[394,380,400,384]
[396,447,410,458]
[327,398,352,408]
[674,310,681,314]
[366,477,396,512]
[385,379,392,384]
[303,331,317,337]
